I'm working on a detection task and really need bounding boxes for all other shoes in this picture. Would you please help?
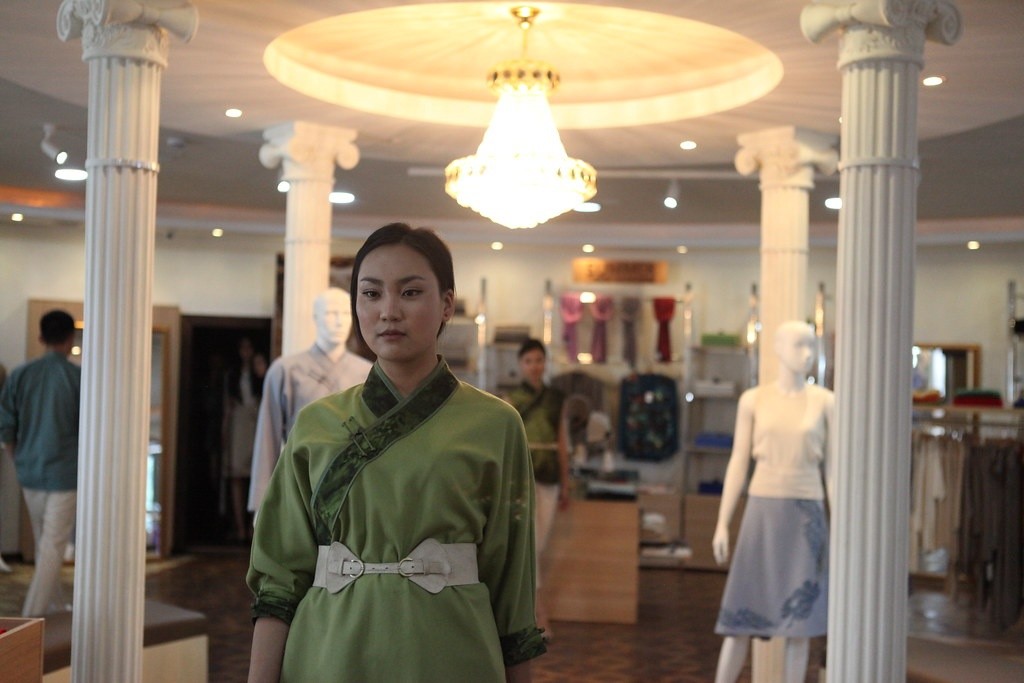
[540,627,553,640]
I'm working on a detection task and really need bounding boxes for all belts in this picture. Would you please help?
[313,538,480,594]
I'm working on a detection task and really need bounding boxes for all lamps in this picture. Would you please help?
[445,7,596,229]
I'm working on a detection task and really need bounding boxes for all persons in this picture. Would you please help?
[1,310,82,618]
[713,321,833,683]
[248,287,372,531]
[245,223,548,683]
[499,340,569,642]
[223,338,267,537]
